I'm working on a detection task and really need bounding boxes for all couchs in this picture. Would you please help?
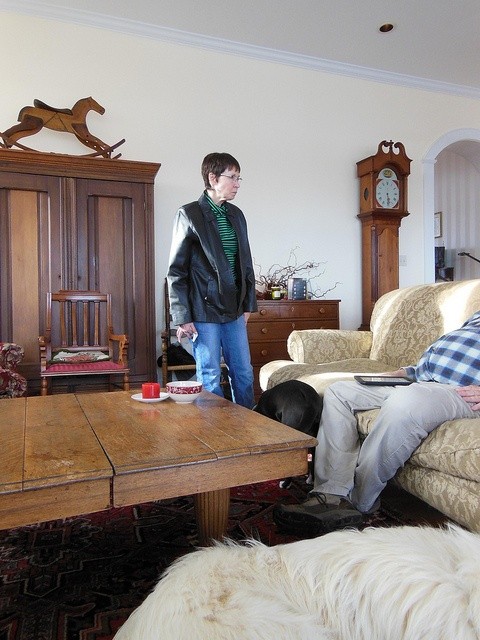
[0,342,28,398]
[259,278,479,531]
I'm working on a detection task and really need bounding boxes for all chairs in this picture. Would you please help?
[38,290,131,396]
[161,277,229,388]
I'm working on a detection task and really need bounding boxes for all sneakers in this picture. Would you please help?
[274,492,362,533]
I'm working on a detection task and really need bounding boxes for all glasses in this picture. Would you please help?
[221,174,243,183]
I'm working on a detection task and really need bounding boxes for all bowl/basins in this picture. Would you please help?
[165,380,203,404]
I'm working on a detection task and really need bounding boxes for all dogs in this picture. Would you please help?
[254,380,322,489]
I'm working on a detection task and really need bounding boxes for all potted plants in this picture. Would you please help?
[252,248,342,299]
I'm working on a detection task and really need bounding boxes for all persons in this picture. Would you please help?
[165,152,258,411]
[273,307,480,536]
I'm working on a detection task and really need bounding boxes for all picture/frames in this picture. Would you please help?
[434,212,442,238]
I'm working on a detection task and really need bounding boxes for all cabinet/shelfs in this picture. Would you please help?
[247,299,341,402]
[1,148,161,397]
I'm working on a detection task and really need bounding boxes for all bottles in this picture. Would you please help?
[271,286,282,299]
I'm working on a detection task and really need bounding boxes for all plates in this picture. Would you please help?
[131,392,169,404]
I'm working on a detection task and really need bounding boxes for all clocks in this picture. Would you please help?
[375,178,400,209]
